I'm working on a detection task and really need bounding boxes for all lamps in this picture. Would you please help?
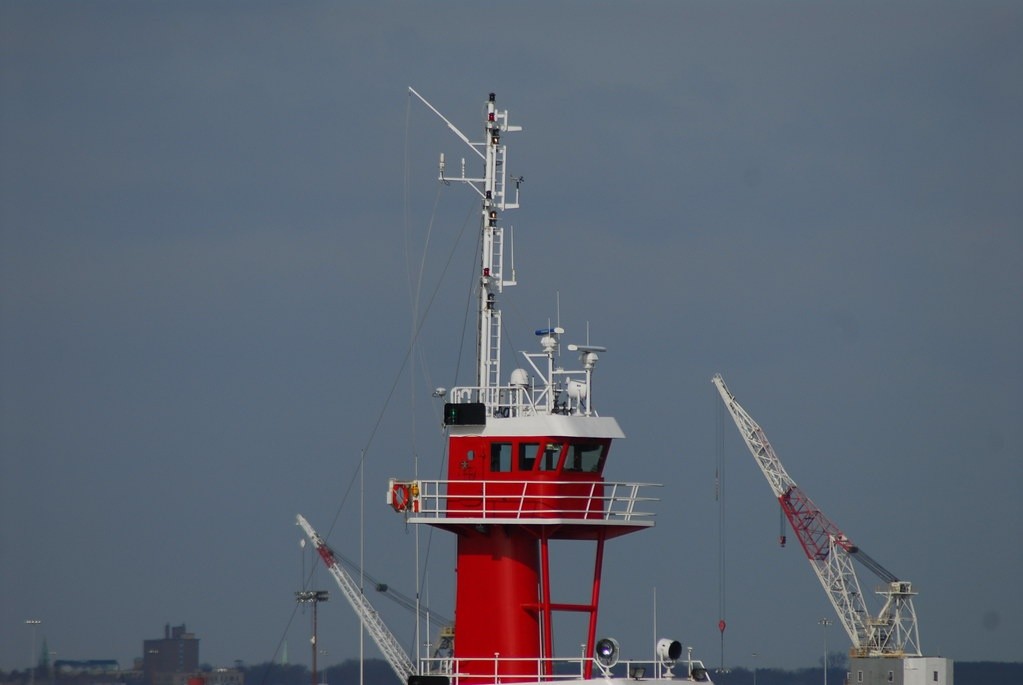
[691,668,707,682]
[656,638,682,680]
[596,638,620,679]
[633,668,645,680]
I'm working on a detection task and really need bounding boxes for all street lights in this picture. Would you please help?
[26,619,43,685]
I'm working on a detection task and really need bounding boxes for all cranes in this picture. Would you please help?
[294,511,419,685]
[709,372,923,672]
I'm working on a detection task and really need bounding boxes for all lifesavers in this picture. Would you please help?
[393,484,409,512]
